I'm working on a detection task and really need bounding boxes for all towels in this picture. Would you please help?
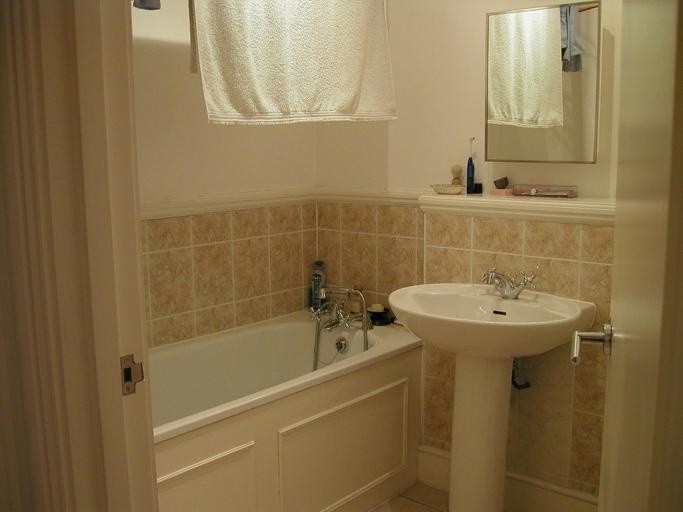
[487,5,565,130]
[186,0,400,128]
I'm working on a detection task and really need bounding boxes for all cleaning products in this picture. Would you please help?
[310,260,326,309]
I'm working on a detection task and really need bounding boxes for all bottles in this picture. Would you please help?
[310,260,326,311]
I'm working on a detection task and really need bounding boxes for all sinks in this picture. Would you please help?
[389,283,595,359]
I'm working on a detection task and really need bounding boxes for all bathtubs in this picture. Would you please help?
[149,310,422,445]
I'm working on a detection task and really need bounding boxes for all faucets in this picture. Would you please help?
[485,269,513,291]
[348,289,368,351]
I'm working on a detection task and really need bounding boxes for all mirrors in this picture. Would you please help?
[484,1,602,165]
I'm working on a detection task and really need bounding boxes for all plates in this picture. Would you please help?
[429,182,466,196]
